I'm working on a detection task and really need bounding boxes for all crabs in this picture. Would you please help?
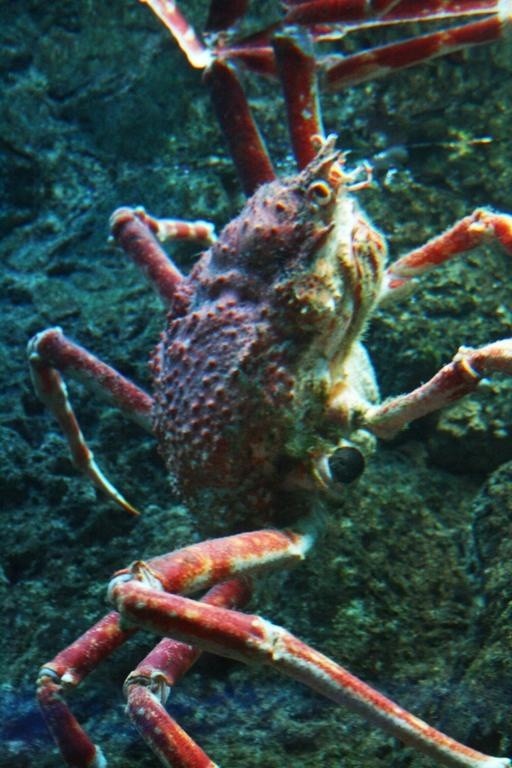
[23,1,512,768]
